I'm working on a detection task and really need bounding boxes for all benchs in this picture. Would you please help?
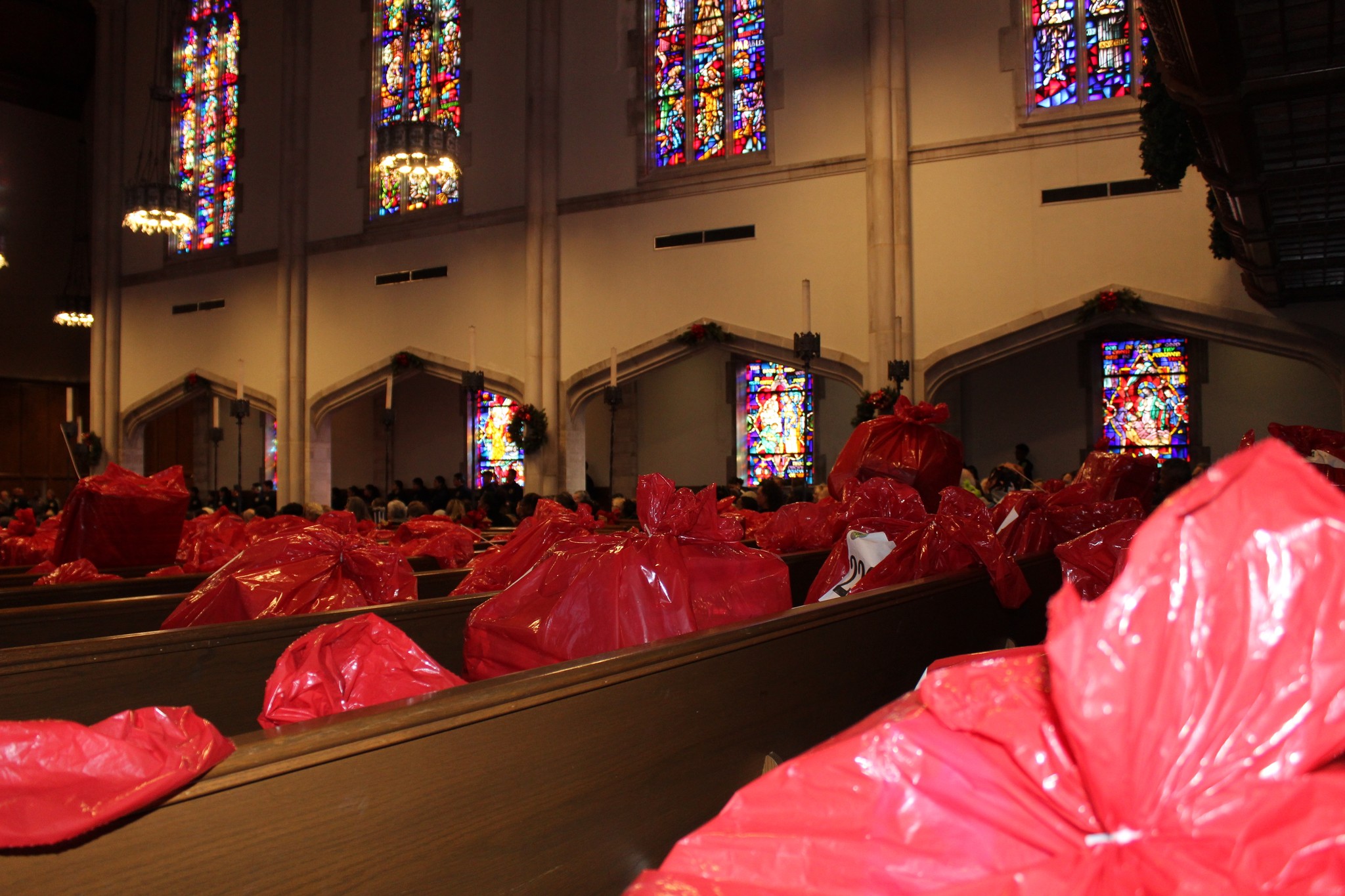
[0,519,1062,896]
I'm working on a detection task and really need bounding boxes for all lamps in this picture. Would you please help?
[51,135,92,329]
[376,0,463,177]
[120,0,196,236]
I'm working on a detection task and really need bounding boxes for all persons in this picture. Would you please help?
[0,470,1053,533]
[1012,442,1038,484]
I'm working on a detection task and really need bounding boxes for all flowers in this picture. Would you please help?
[691,324,706,338]
[866,388,891,409]
[396,354,410,367]
[1097,291,1120,314]
[515,404,531,421]
[187,373,198,385]
[82,433,92,446]
